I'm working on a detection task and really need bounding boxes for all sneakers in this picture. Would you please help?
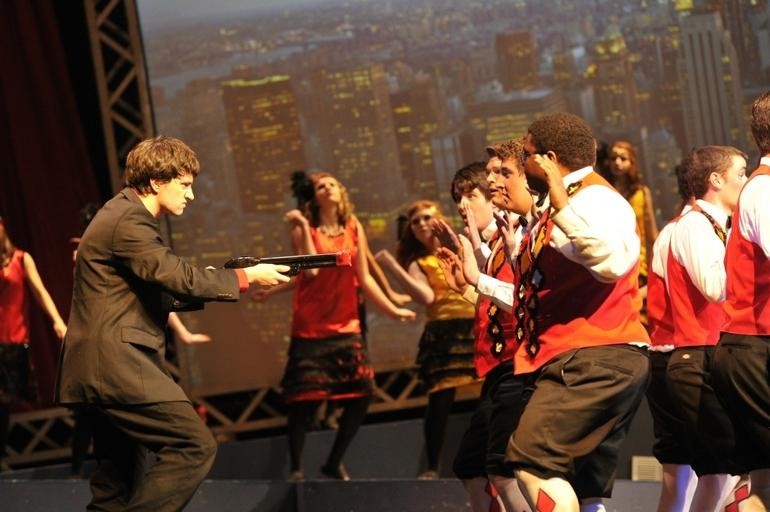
[321,463,351,481]
[287,469,304,481]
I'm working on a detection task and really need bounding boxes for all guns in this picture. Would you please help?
[224,251,352,276]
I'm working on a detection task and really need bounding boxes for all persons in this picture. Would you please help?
[0,217,213,460]
[251,169,478,481]
[54,136,291,512]
[433,93,770,512]
